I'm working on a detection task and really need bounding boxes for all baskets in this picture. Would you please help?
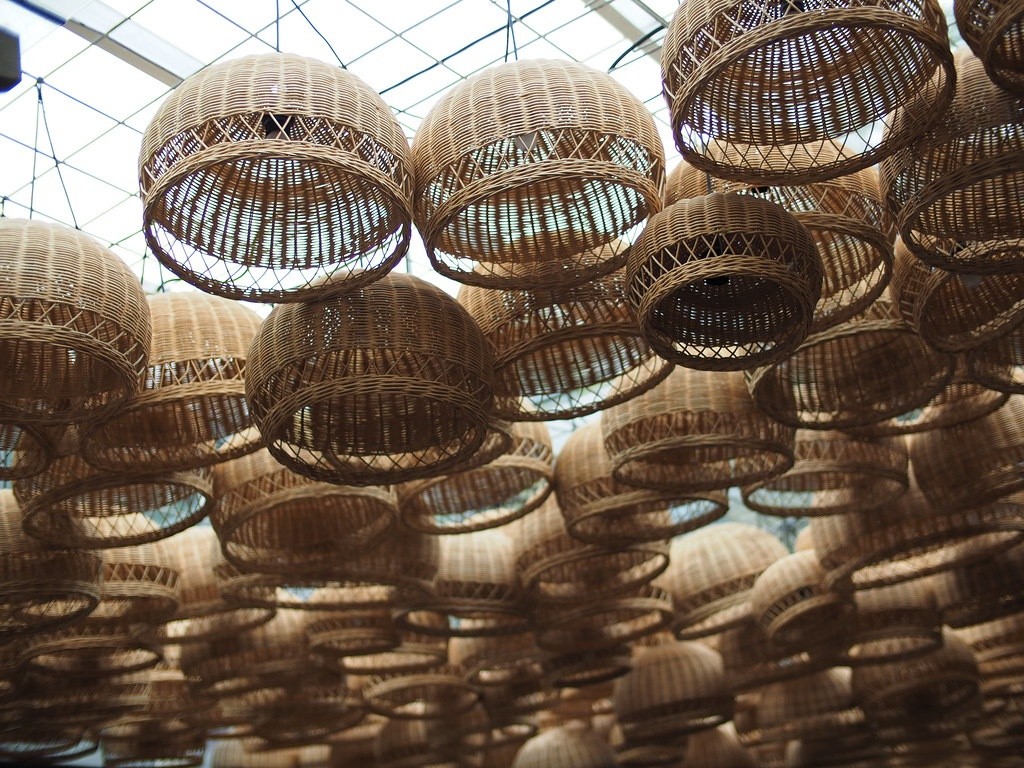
[0,0,1024,768]
